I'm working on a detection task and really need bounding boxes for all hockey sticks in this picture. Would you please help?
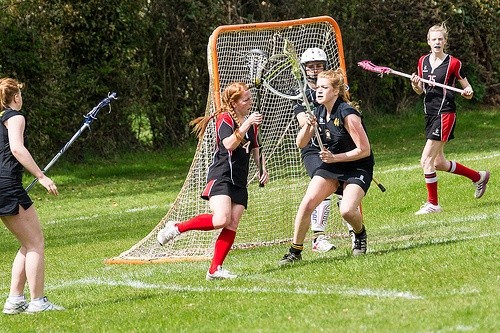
[358,60,465,93]
[262,54,387,193]
[245,49,269,188]
[283,39,325,151]
[26,91,117,191]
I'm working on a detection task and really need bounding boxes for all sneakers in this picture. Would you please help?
[414,201,441,216]
[3,295,30,315]
[157,220,181,246]
[27,296,65,312]
[312,234,336,253]
[279,252,303,264]
[206,265,238,282]
[473,170,491,199]
[348,224,368,256]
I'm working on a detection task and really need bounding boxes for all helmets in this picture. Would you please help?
[300,47,327,65]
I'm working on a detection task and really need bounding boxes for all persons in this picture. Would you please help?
[409,25,490,216]
[155,82,271,282]
[0,76,67,316]
[279,47,376,265]
